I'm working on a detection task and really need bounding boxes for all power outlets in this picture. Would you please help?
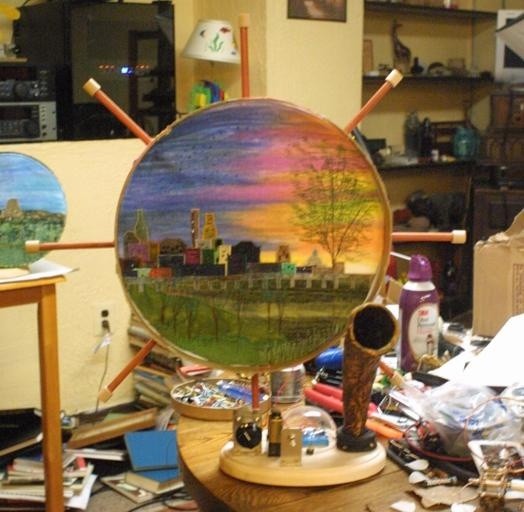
[92,303,115,335]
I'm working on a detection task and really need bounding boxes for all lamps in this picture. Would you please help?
[180,19,240,104]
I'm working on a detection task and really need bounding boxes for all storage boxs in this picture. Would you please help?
[473,208,524,340]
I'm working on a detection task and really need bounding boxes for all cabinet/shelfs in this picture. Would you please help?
[473,95,524,256]
[361,1,498,320]
[0,274,67,512]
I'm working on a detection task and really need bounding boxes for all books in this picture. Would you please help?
[0,406,186,512]
[128,311,182,410]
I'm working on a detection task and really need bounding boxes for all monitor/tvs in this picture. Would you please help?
[494,8,524,84]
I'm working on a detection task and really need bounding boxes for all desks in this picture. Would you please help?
[176,370,524,511]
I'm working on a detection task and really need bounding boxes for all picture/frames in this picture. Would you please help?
[288,0,347,23]
